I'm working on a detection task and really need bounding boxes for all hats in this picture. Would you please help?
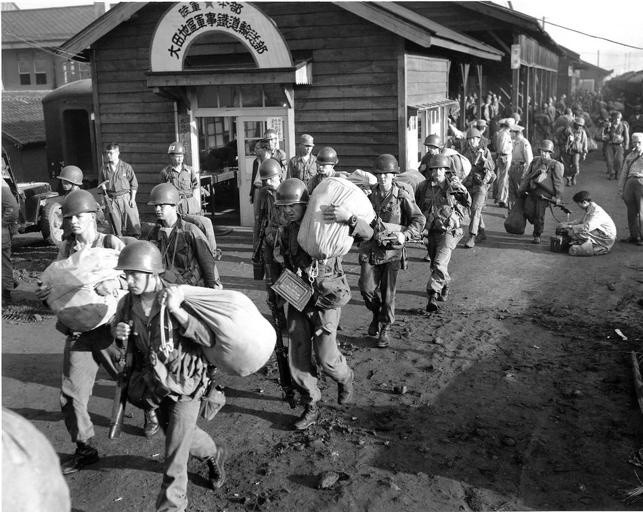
[299,134,314,146]
[477,113,527,135]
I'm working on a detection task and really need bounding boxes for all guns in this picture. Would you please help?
[378,230,433,246]
[524,191,571,215]
[110,294,133,439]
[261,252,296,410]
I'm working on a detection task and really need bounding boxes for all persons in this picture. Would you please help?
[36,190,165,477]
[139,183,223,291]
[159,141,203,217]
[109,238,225,510]
[596,92,642,244]
[55,166,101,241]
[414,91,617,312]
[2,170,18,300]
[249,128,427,429]
[99,144,139,234]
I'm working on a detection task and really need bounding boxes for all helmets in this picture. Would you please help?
[423,130,482,168]
[315,146,337,165]
[167,142,186,155]
[543,95,625,126]
[537,140,555,153]
[62,191,97,215]
[272,179,310,206]
[113,241,165,274]
[147,183,179,207]
[262,129,276,140]
[567,177,576,186]
[259,159,282,179]
[371,153,400,174]
[57,165,84,185]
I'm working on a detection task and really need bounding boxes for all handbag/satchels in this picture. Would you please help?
[504,193,527,233]
[271,269,315,312]
[308,273,352,308]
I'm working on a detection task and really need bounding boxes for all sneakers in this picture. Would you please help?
[607,173,621,180]
[622,237,643,245]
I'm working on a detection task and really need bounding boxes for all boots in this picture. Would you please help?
[58,446,99,475]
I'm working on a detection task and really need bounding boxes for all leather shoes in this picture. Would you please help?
[144,407,162,435]
[295,406,318,428]
[426,286,449,310]
[532,236,541,244]
[336,370,355,404]
[206,445,226,486]
[465,229,487,248]
[368,314,390,347]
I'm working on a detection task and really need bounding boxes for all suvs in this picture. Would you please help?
[1,146,64,247]
